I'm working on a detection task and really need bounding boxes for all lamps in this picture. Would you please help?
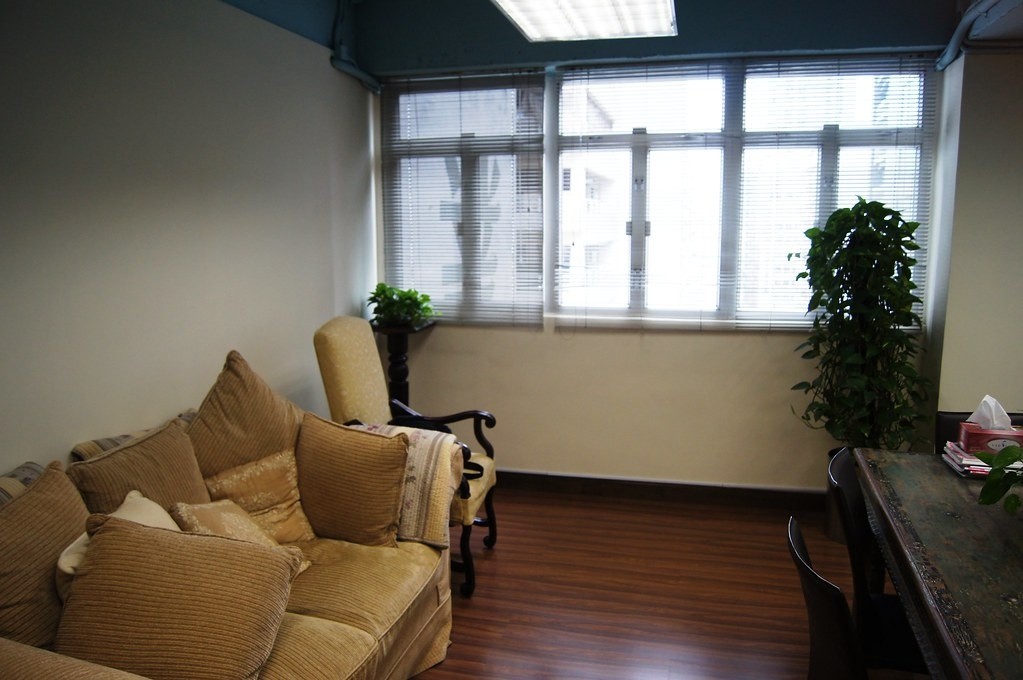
[492,0,677,44]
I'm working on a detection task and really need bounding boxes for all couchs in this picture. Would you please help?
[0,407,464,680]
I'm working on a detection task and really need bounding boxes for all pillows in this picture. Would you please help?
[55,489,182,604]
[53,513,304,680]
[64,419,211,512]
[204,446,315,543]
[296,411,411,549]
[186,350,306,479]
[169,497,312,577]
[0,460,91,652]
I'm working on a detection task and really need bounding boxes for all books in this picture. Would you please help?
[942,441,1023,479]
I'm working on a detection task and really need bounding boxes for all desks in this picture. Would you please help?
[851,447,1023,680]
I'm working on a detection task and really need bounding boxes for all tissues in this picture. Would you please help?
[957,394,1023,454]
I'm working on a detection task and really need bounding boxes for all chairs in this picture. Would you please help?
[827,443,929,675]
[934,411,1023,453]
[786,515,870,680]
[313,316,498,600]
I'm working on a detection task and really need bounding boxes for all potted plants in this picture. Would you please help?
[785,196,937,545]
[366,284,433,328]
